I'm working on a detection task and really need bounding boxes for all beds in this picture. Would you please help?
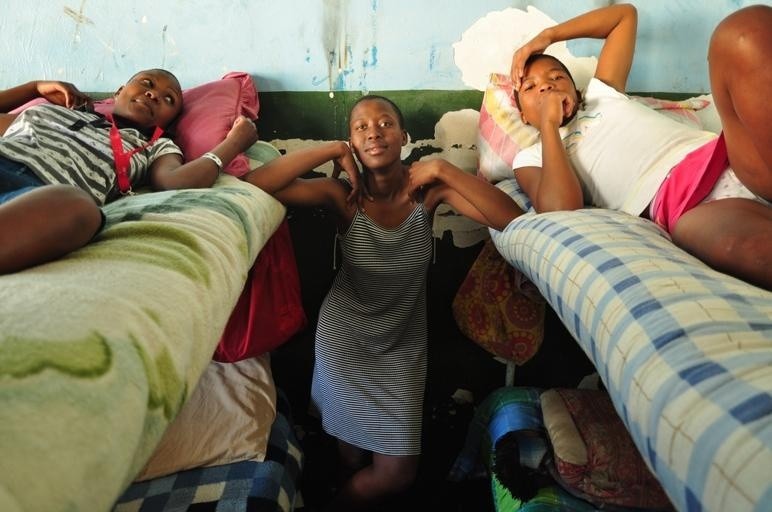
[482,172,771,509]
[2,136,289,511]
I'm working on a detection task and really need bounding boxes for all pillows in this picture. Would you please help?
[4,70,262,180]
[474,72,711,185]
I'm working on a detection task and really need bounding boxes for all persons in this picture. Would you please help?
[508,3,769,292]
[1,68,260,276]
[238,96,528,511]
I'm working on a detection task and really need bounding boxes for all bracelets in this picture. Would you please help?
[202,149,224,175]
